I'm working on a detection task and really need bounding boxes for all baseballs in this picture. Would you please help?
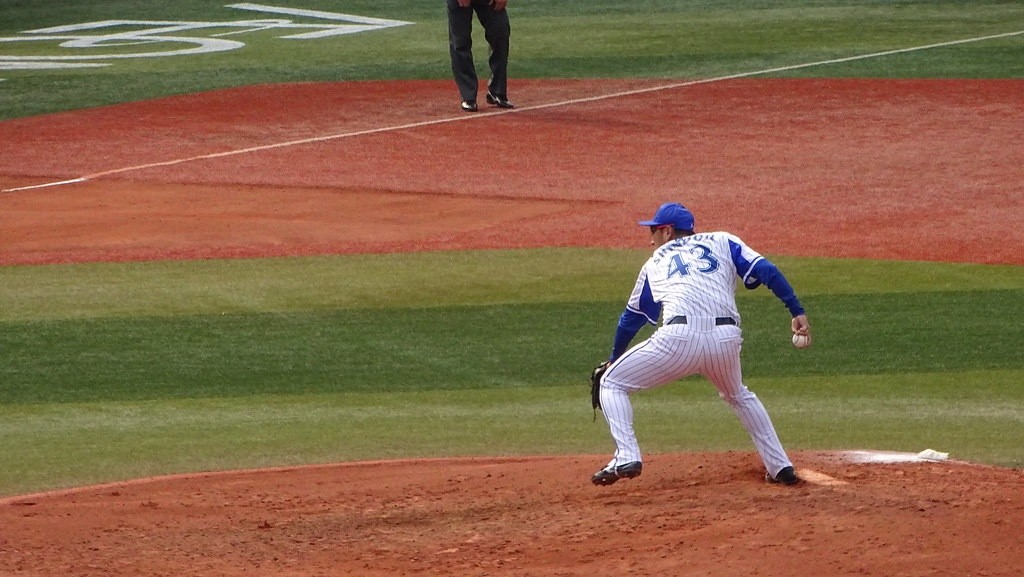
[792,333,809,348]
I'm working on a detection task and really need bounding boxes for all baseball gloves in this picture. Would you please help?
[587,360,610,423]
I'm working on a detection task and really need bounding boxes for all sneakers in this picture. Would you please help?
[591,461,642,486]
[486,93,515,110]
[765,466,796,484]
[462,98,478,113]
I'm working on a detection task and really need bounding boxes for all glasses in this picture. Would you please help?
[650,223,676,233]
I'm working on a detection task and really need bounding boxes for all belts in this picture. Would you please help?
[668,316,736,325]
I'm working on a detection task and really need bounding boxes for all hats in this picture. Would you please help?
[638,202,693,231]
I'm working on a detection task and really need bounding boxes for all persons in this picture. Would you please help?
[447,0,514,111]
[590,202,812,486]
